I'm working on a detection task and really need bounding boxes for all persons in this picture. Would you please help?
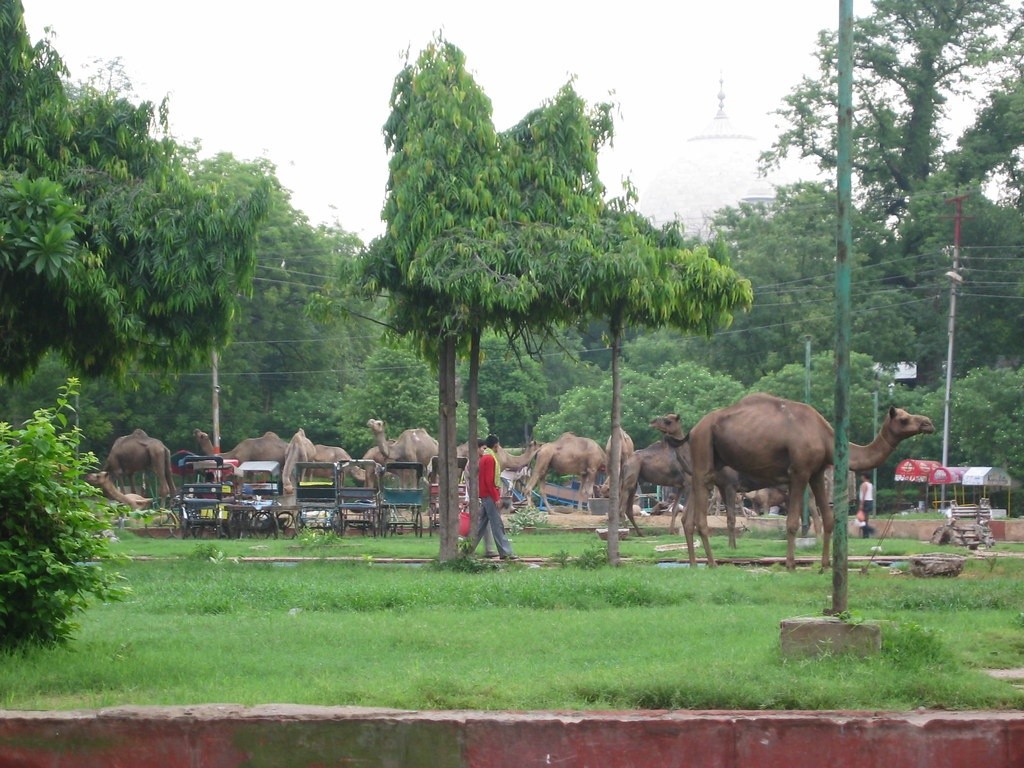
[857,472,874,539]
[464,433,519,559]
[195,471,217,498]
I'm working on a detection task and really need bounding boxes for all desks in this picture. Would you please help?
[225,505,303,540]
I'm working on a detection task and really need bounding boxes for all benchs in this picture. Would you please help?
[178,483,468,512]
[951,506,991,520]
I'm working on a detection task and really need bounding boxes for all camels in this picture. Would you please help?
[85,390,935,572]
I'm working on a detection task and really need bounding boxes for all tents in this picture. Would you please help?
[894,459,1011,486]
